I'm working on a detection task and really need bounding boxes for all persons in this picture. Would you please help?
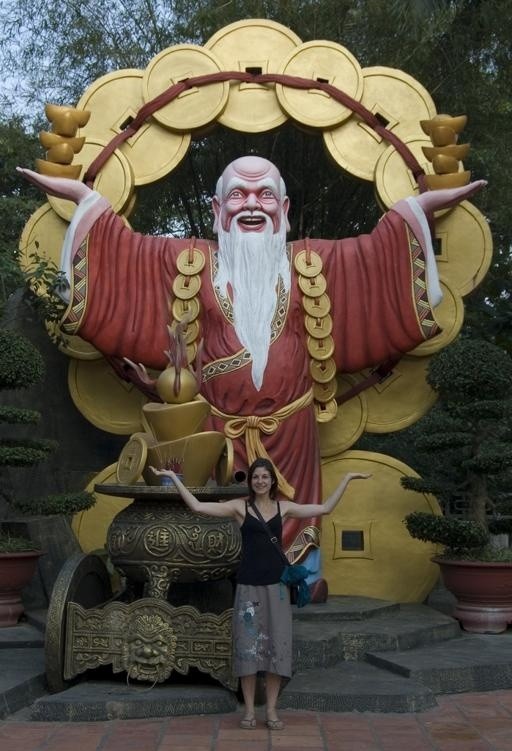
[146,458,374,732]
[15,155,491,602]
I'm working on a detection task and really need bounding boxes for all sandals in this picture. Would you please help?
[238,718,256,731]
[265,718,284,731]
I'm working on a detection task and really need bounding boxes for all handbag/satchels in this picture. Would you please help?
[284,563,311,608]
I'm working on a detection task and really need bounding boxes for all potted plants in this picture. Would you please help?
[402,337,511,635]
[0,328,99,629]
